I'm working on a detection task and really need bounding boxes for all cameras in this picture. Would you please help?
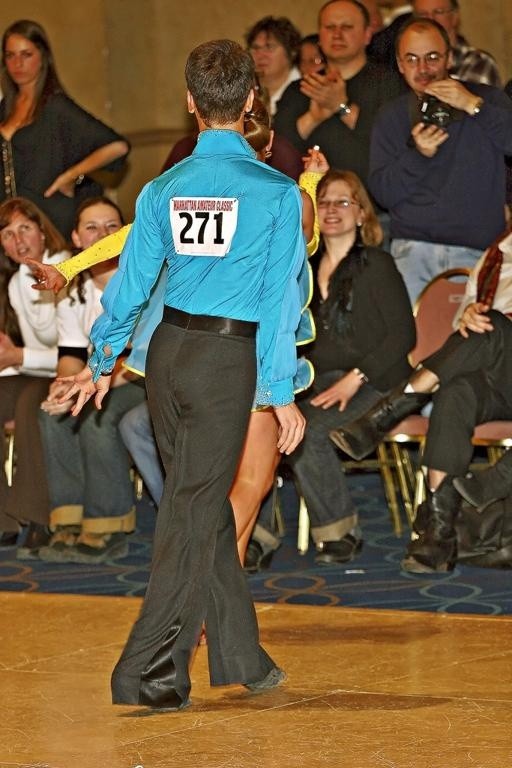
[420,92,451,127]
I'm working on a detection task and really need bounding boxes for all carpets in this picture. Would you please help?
[1,468,512,615]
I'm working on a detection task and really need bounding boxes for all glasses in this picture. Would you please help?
[317,199,361,205]
[402,48,450,67]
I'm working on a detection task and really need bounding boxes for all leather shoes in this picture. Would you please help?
[242,539,273,573]
[315,529,363,566]
[248,667,285,690]
[16,522,129,565]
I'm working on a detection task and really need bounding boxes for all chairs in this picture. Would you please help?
[109,355,144,501]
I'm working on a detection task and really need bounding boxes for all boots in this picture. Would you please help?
[402,446,511,576]
[330,360,440,461]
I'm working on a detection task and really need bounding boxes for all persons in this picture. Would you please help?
[2,0,511,579]
[55,40,312,711]
